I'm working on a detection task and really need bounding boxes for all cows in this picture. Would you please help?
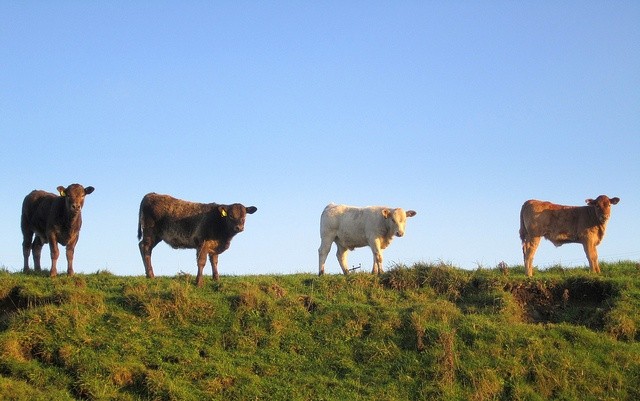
[318,202,417,277]
[21,184,95,278]
[137,192,257,288]
[519,195,620,277]
